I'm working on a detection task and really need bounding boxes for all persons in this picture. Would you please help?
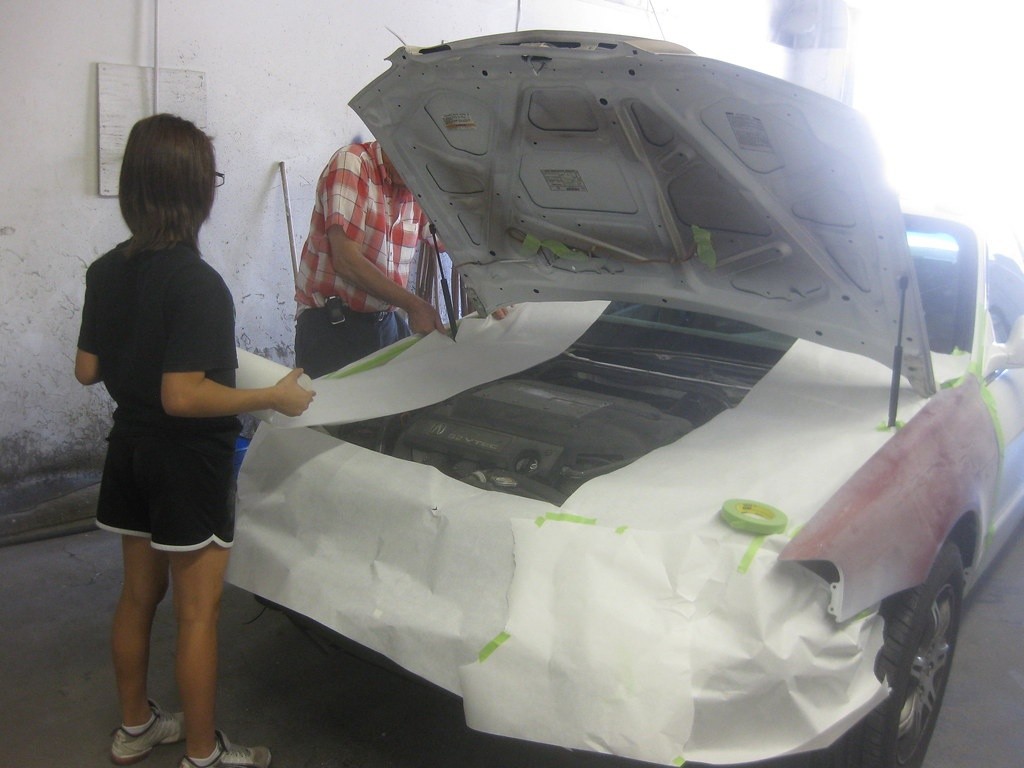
[75,113,315,767]
[293,139,517,380]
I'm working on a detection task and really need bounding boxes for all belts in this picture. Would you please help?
[345,307,390,323]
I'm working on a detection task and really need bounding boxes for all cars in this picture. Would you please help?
[218,24,1023,767]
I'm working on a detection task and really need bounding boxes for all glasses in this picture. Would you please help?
[215,171,225,187]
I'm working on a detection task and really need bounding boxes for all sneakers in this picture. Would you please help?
[109,695,187,766]
[180,728,271,768]
